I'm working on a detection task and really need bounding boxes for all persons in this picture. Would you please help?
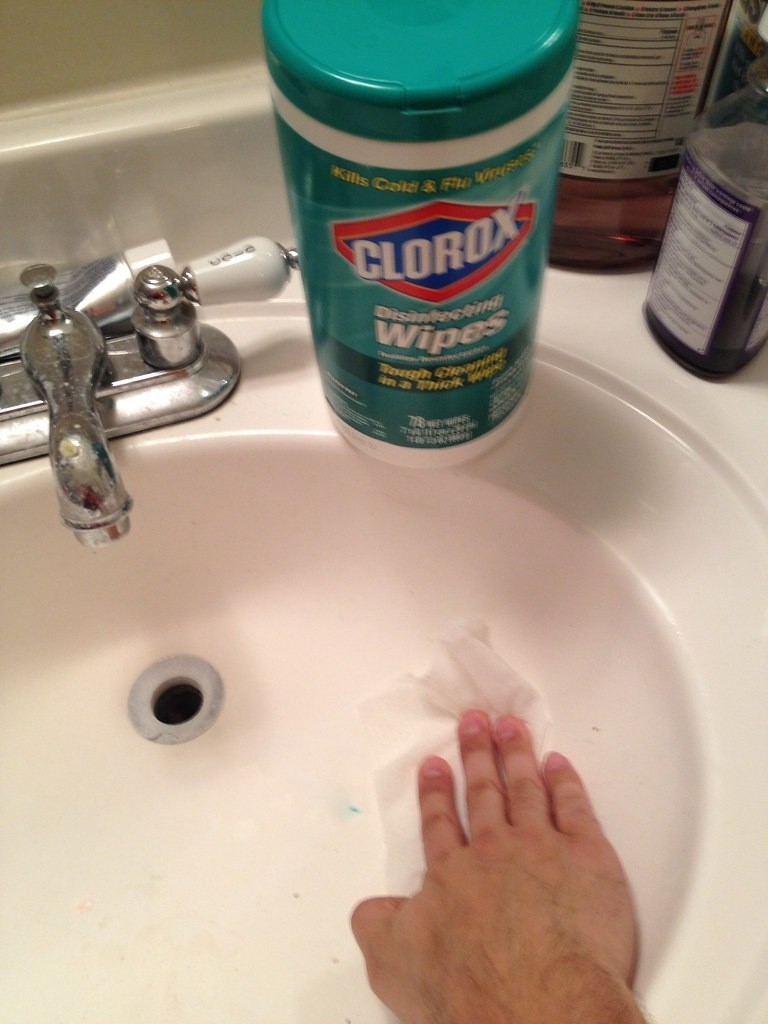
[350,708,648,1024]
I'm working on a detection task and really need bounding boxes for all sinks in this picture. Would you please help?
[0,296,768,1023]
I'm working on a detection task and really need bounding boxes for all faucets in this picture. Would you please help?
[0,236,304,553]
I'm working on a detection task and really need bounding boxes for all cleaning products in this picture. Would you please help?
[546,0,766,384]
[258,0,581,473]
